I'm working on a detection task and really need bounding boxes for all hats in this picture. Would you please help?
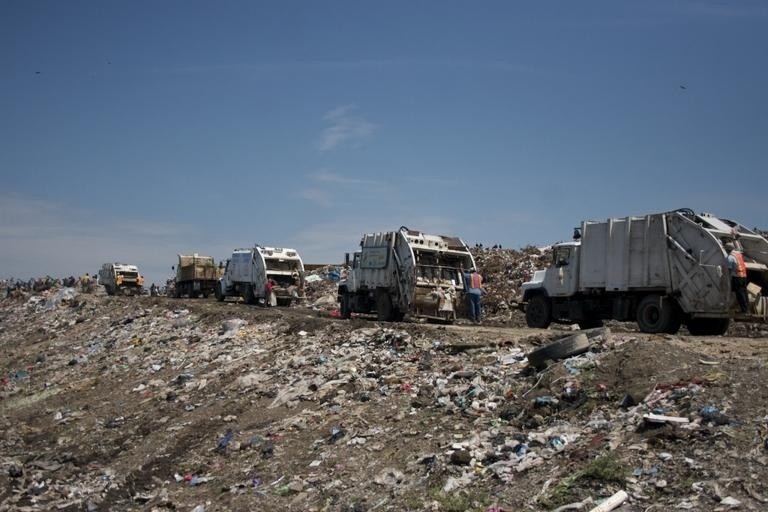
[723,243,733,249]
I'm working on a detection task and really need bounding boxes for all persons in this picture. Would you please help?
[265,278,272,308]
[291,269,300,286]
[444,286,458,322]
[151,280,175,297]
[723,242,749,312]
[2,273,98,292]
[460,266,486,325]
[115,273,124,287]
[136,275,144,289]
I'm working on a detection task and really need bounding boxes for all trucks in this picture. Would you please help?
[509,208,767,333]
[337,227,478,321]
[97,263,144,295]
[215,244,308,306]
[166,253,225,298]
